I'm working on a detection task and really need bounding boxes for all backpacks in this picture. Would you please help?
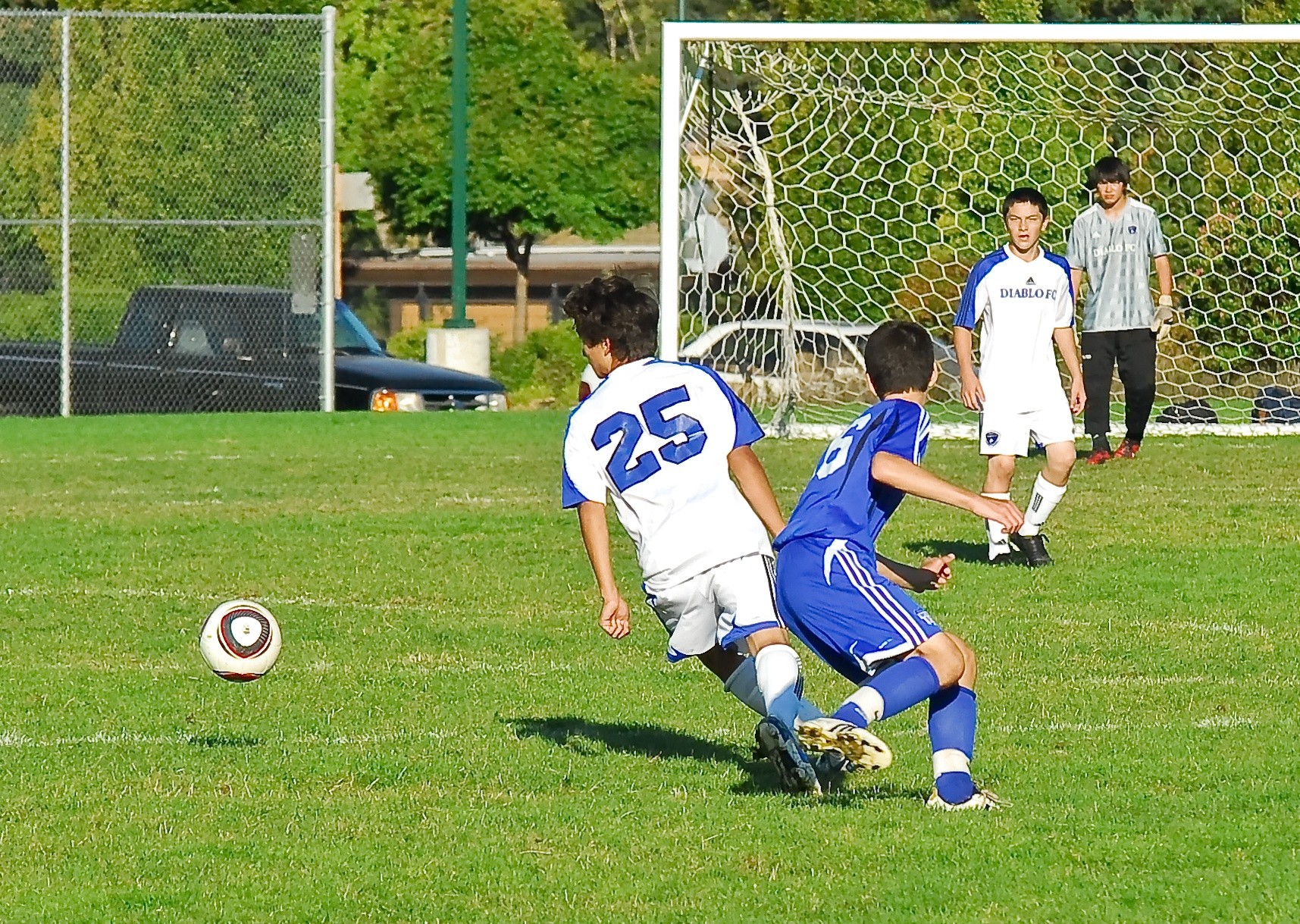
[1254,386,1299,424]
[1158,397,1217,424]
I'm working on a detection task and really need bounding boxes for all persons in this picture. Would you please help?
[951,184,1089,569]
[1064,157,1177,468]
[772,320,1014,814]
[559,274,892,797]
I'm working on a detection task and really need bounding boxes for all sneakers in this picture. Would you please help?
[1115,438,1142,461]
[922,786,1007,815]
[750,715,825,797]
[798,721,893,774]
[1010,531,1052,568]
[986,553,1012,569]
[1085,448,1111,464]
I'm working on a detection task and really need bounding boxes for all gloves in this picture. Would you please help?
[1152,294,1172,338]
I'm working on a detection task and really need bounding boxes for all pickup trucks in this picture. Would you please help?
[2,285,510,414]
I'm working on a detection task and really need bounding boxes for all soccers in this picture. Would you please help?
[197,599,283,683]
